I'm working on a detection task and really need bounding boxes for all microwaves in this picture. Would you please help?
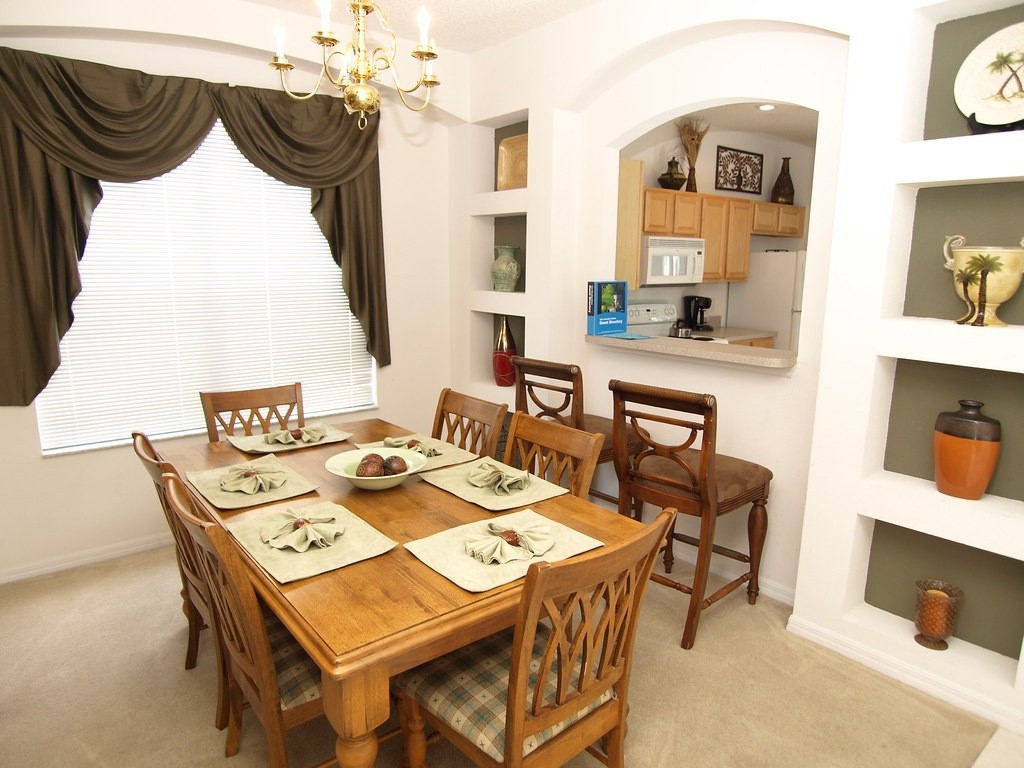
[639,235,705,287]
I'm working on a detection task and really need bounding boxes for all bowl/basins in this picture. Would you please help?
[325,447,428,491]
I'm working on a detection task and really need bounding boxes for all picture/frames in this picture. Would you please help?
[714,144,764,195]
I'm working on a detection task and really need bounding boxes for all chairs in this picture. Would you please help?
[159,472,405,767]
[608,380,773,651]
[609,381,775,650]
[509,354,651,522]
[130,432,229,733]
[200,384,305,441]
[389,506,679,768]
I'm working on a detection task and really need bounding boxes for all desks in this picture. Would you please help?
[155,415,653,768]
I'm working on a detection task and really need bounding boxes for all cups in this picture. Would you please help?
[942,235,1024,327]
[914,579,963,651]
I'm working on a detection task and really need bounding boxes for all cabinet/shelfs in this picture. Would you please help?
[846,4,1024,694]
[643,188,805,282]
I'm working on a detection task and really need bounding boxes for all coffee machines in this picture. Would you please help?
[684,295,714,331]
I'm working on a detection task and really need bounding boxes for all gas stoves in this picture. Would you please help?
[626,304,729,345]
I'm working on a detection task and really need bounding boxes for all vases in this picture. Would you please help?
[684,168,698,192]
[491,245,520,294]
[492,313,519,387]
[770,156,795,206]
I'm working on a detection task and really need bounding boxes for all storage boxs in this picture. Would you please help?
[586,281,628,336]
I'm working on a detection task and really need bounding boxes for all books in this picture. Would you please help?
[587,281,628,336]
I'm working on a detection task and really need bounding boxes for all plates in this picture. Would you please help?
[496,133,527,191]
[953,22,1024,126]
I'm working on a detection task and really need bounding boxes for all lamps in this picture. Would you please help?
[274,3,437,121]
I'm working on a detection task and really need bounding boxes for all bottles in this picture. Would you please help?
[932,400,1001,501]
[771,157,794,205]
[493,315,517,387]
[491,245,522,292]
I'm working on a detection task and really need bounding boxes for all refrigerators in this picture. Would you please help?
[726,249,807,352]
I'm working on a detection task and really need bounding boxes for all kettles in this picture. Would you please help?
[674,319,692,338]
